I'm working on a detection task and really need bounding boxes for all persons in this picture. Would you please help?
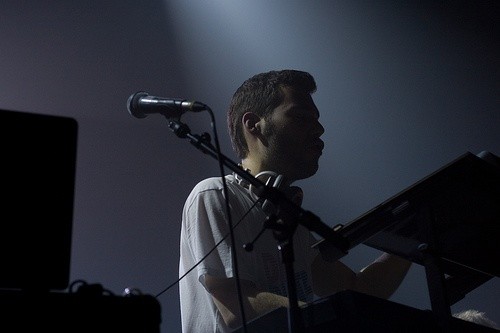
[179,69,412,333]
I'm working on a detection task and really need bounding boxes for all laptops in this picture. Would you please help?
[1,109,80,290]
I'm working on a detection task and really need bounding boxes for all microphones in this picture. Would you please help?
[125,92,209,119]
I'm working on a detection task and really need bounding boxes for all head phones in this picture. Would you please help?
[233,169,283,209]
